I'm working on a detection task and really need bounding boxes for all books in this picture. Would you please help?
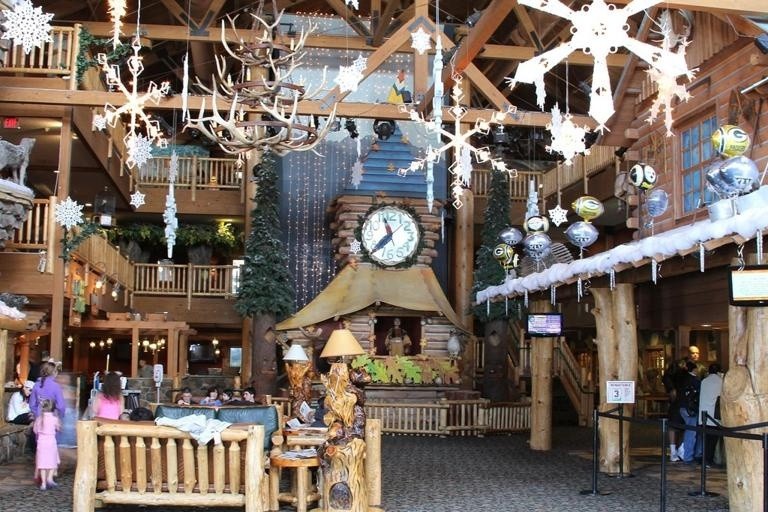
[280,448,318,459]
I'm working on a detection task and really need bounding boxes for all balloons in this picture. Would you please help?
[492,123,759,271]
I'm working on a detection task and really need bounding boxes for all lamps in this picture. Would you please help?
[187,111,599,153]
[281,344,310,364]
[577,77,595,98]
[101,271,121,298]
[440,10,484,68]
[75,260,109,290]
[318,328,367,364]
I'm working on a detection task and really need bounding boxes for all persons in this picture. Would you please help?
[3,356,263,490]
[385,318,411,355]
[308,397,327,427]
[661,344,725,463]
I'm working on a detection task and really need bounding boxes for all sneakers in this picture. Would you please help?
[40,483,46,490]
[670,454,679,461]
[678,448,685,459]
[47,481,58,488]
[33,478,41,486]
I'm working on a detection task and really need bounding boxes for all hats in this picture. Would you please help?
[686,361,697,372]
[24,381,34,387]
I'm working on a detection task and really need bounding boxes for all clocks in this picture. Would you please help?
[356,200,427,270]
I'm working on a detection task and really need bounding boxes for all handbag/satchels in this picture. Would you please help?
[713,440,726,465]
[714,396,721,419]
[685,385,699,416]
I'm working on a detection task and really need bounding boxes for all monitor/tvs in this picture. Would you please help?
[726,264,768,308]
[188,341,218,363]
[526,312,564,335]
